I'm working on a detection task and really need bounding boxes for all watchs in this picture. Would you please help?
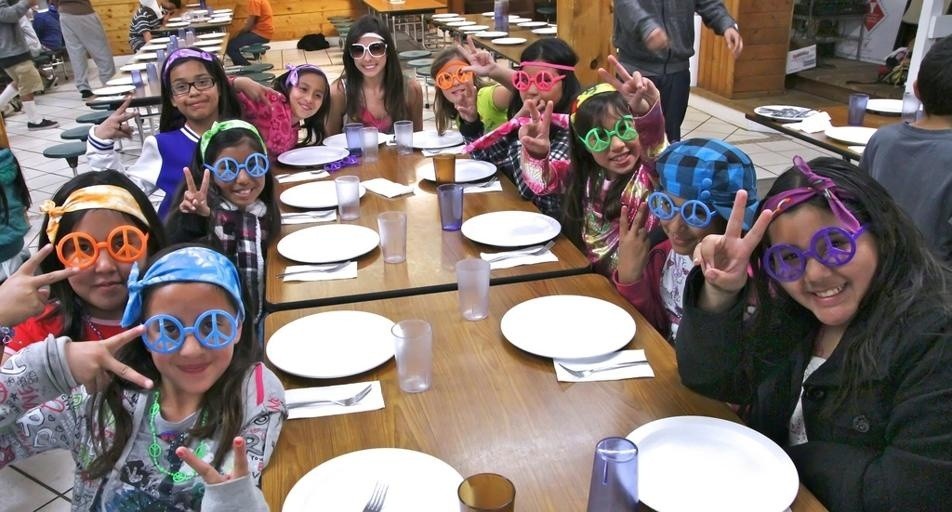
[731,23,739,31]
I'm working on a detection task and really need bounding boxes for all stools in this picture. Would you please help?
[60,126,94,145]
[417,66,440,108]
[91,105,121,151]
[246,43,263,63]
[240,46,267,64]
[427,79,440,114]
[340,32,349,52]
[408,58,436,108]
[399,50,433,83]
[336,27,351,48]
[537,6,556,26]
[240,63,273,73]
[77,111,121,154]
[242,72,275,91]
[331,19,352,25]
[334,23,350,47]
[31,55,50,95]
[328,16,353,21]
[43,142,87,177]
[49,46,71,83]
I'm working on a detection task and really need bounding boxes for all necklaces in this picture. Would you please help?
[148,386,211,483]
[83,314,103,339]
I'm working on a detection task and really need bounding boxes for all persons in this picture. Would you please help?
[322,15,423,137]
[127,1,181,53]
[17,13,42,59]
[432,35,518,145]
[31,5,63,93]
[611,139,776,424]
[86,45,242,221]
[0,241,288,512]
[676,156,952,511]
[856,34,952,265]
[226,0,273,67]
[518,54,670,277]
[227,62,331,161]
[0,0,58,131]
[54,0,115,100]
[468,37,582,217]
[610,0,743,144]
[0,169,162,366]
[161,119,282,334]
[0,144,32,262]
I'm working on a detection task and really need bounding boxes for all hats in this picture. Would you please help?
[655,137,760,232]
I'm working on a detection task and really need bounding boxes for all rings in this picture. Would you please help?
[119,123,121,130]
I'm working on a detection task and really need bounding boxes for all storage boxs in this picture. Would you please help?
[785,42,818,75]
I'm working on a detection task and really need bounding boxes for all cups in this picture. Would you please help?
[156,50,164,62]
[457,473,516,512]
[437,183,463,231]
[392,318,433,394]
[587,436,638,512]
[377,210,407,264]
[847,93,869,126]
[394,120,413,155]
[131,70,143,88]
[168,43,173,55]
[457,257,490,321]
[343,123,363,157]
[361,127,378,162]
[146,63,158,83]
[179,29,185,39]
[178,40,185,48]
[170,35,178,50]
[335,175,360,224]
[900,91,921,124]
[434,153,457,188]
[186,32,193,44]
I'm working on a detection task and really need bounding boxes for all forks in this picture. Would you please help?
[559,361,649,378]
[278,207,334,220]
[486,240,556,263]
[362,480,388,511]
[283,383,375,411]
[276,260,352,278]
[454,176,498,188]
[424,147,456,154]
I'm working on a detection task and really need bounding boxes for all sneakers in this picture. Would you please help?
[27,119,59,131]
[82,92,94,101]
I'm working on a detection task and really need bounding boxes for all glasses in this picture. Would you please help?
[143,309,243,354]
[350,40,388,61]
[433,65,473,90]
[647,192,721,228]
[573,114,638,153]
[204,152,270,183]
[510,70,566,92]
[171,76,218,96]
[763,221,875,284]
[54,224,150,270]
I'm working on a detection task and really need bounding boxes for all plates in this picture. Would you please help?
[140,44,167,50]
[190,18,211,22]
[208,17,232,23]
[508,18,532,24]
[276,224,379,263]
[277,145,348,165]
[432,14,459,18]
[210,13,233,17]
[616,415,800,512]
[461,211,562,246]
[531,28,557,34]
[322,132,386,150]
[197,33,226,38]
[213,9,232,13]
[120,63,146,71]
[267,310,405,375]
[150,37,170,43]
[402,130,463,148]
[106,77,133,85]
[418,158,497,183]
[482,12,494,16]
[165,22,189,27]
[500,294,636,360]
[200,47,221,52]
[508,15,521,18]
[193,40,223,45]
[92,85,136,95]
[433,19,465,22]
[824,126,879,145]
[446,22,476,26]
[459,26,489,30]
[280,180,366,209]
[138,52,167,59]
[517,22,548,26]
[858,98,927,113]
[753,104,821,120]
[491,37,527,44]
[168,17,182,21]
[282,448,472,512]
[475,31,508,37]
